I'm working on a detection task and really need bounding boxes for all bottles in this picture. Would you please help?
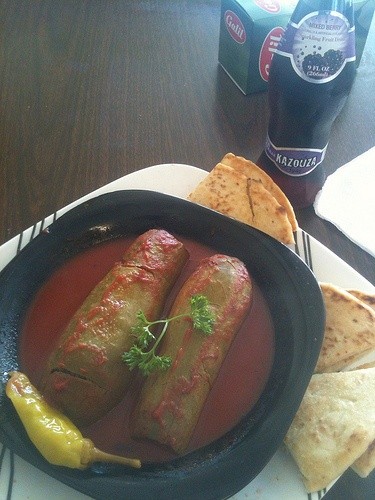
[256,0,356,209]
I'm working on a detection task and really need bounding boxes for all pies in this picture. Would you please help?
[282,280,375,493]
[185,153,300,245]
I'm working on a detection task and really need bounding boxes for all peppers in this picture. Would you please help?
[5,370,142,470]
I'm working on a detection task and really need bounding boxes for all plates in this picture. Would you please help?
[1,164,374,499]
[0,188,325,500]
